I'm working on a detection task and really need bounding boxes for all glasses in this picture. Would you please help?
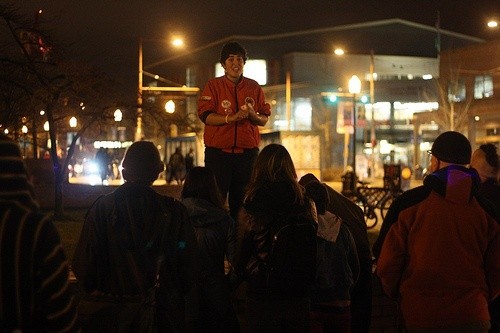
[427,150,432,155]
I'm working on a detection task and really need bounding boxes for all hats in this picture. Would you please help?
[121,141,164,178]
[431,131,471,164]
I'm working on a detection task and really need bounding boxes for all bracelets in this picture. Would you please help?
[225,114,230,124]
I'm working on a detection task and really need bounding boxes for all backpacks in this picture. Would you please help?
[260,214,317,297]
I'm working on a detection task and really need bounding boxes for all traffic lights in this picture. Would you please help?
[356,95,371,105]
[322,95,339,104]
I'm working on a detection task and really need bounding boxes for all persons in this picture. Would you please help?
[383,149,402,165]
[0,128,83,333]
[197,40,271,208]
[73,130,499,333]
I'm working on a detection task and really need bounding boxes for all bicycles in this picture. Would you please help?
[96,160,109,183]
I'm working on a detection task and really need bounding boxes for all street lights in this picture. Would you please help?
[43,121,50,151]
[113,108,123,160]
[347,74,362,176]
[163,100,176,166]
[69,116,78,139]
[21,125,29,159]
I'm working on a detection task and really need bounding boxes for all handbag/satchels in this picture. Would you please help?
[78,287,156,333]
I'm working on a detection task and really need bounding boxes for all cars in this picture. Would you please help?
[67,156,123,180]
